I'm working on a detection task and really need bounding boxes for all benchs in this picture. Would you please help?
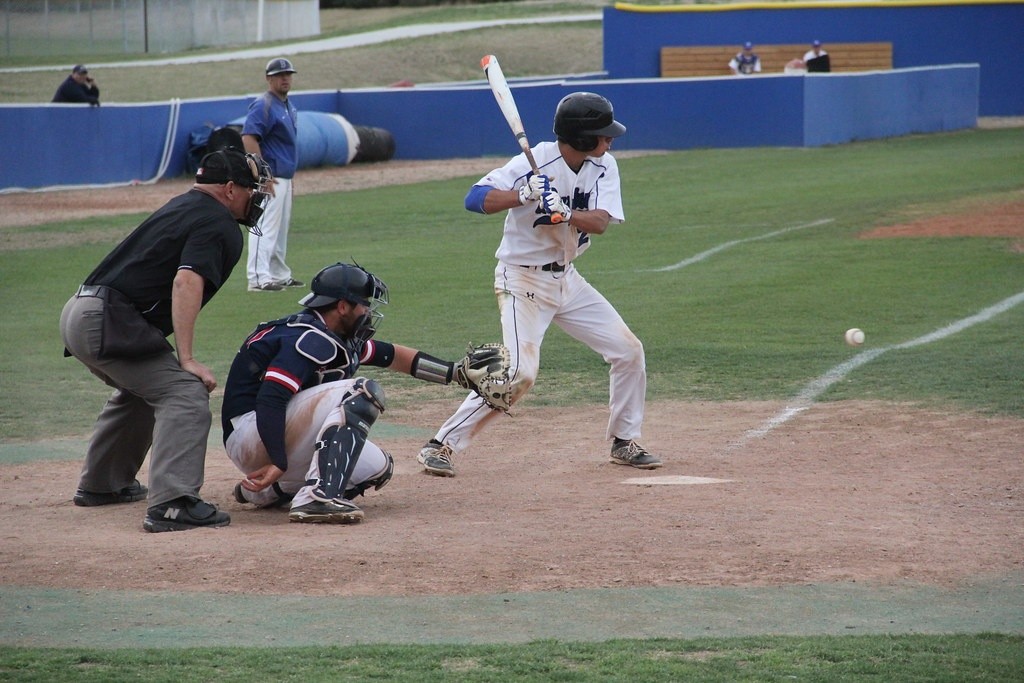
[660,42,892,77]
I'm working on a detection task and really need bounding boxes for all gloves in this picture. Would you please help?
[539,190,572,222]
[519,174,555,206]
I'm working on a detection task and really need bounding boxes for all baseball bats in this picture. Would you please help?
[480,54,562,224]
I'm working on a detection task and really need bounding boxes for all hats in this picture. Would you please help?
[196,149,260,191]
[73,65,87,73]
[813,40,819,45]
[743,40,752,48]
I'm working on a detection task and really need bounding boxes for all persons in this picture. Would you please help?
[51,64,100,106]
[221,255,512,524]
[803,40,830,72]
[417,91,664,477]
[239,57,305,291]
[728,41,761,75]
[59,147,272,533]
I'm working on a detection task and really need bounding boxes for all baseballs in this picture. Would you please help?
[846,328,865,347]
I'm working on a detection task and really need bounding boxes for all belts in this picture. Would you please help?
[75,285,104,299]
[520,261,570,274]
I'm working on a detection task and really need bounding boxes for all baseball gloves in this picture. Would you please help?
[458,342,512,415]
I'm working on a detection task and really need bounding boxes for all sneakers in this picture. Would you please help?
[416,439,455,477]
[143,494,231,533]
[234,481,248,503]
[608,439,663,468]
[249,282,285,292]
[288,493,364,523]
[281,279,306,288]
[73,480,149,506]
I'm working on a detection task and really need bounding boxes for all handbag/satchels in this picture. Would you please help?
[97,290,177,359]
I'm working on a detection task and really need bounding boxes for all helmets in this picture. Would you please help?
[296,263,380,308]
[552,92,627,152]
[265,58,297,76]
